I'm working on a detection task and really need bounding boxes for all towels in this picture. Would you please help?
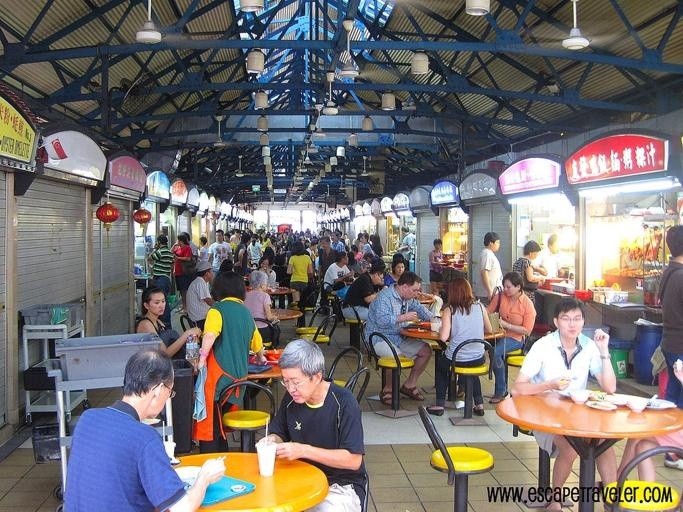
[191,368,207,423]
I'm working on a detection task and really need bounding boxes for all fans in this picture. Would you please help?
[235,155,245,178]
[299,150,308,172]
[303,138,314,165]
[313,105,326,138]
[296,166,304,181]
[322,72,338,115]
[213,115,226,147]
[308,124,318,153]
[135,0,161,45]
[561,0,590,50]
[339,20,359,78]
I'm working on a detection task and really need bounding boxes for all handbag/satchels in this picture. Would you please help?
[488,286,502,333]
[175,251,196,267]
[522,277,538,290]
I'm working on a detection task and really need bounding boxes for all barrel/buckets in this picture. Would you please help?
[608,349,629,380]
[633,326,665,387]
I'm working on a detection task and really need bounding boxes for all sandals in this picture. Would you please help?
[491,392,510,404]
[379,390,393,405]
[546,498,563,512]
[400,384,425,401]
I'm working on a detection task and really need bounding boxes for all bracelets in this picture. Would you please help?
[600,354,611,359]
[507,323,511,331]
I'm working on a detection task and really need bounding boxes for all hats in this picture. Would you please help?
[196,262,213,271]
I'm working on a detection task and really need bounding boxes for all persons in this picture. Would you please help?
[224,233,237,251]
[212,259,246,301]
[257,256,277,286]
[534,234,565,277]
[658,225,683,410]
[258,247,273,268]
[371,234,385,257]
[147,235,176,329]
[429,238,446,297]
[318,236,339,315]
[365,272,435,406]
[384,259,407,287]
[426,278,492,416]
[232,227,330,242]
[208,230,232,272]
[186,261,216,330]
[617,359,683,485]
[197,237,208,271]
[513,240,548,322]
[511,298,617,512]
[181,232,198,266]
[272,255,287,282]
[192,272,267,454]
[330,227,359,252]
[487,272,536,404]
[476,232,505,308]
[247,234,263,267]
[286,242,312,302]
[324,252,355,294]
[359,233,373,259]
[136,286,201,358]
[244,271,280,350]
[64,347,226,512]
[170,234,193,313]
[234,234,251,277]
[306,244,318,261]
[341,267,384,319]
[256,339,368,512]
[397,226,412,260]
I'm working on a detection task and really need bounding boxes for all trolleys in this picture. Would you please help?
[44,357,178,512]
[22,319,92,427]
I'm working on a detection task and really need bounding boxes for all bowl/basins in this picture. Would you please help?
[628,398,647,414]
[569,390,589,404]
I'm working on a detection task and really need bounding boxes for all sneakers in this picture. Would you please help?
[664,459,683,472]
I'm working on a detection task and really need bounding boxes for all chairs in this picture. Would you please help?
[507,336,530,356]
[180,315,193,332]
[505,355,526,367]
[327,345,363,392]
[338,300,365,352]
[368,331,418,419]
[343,366,370,403]
[254,317,275,349]
[418,405,495,512]
[295,306,330,335]
[300,314,337,346]
[217,381,275,453]
[322,281,332,294]
[448,339,494,427]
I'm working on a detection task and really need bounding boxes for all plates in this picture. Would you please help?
[606,393,632,407]
[170,460,181,464]
[558,390,570,398]
[586,400,617,411]
[645,398,677,411]
[171,465,256,507]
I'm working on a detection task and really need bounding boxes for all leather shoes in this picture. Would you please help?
[473,407,484,416]
[426,406,445,416]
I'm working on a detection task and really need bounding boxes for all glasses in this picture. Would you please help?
[281,376,311,387]
[556,317,582,323]
[152,382,176,398]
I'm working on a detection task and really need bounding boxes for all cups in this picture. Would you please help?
[255,442,277,477]
[164,441,175,459]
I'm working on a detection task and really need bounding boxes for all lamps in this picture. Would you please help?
[349,132,358,146]
[325,164,331,172]
[259,133,269,145]
[246,48,264,74]
[382,90,396,110]
[330,157,337,165]
[320,170,325,177]
[254,89,269,110]
[264,157,271,166]
[239,0,265,13]
[257,116,269,131]
[411,50,429,75]
[362,115,374,130]
[465,0,491,16]
[337,146,345,157]
[262,146,270,157]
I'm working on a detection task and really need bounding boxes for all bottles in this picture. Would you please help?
[192,335,200,359]
[186,337,194,359]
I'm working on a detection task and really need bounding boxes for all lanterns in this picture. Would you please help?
[96,202,120,232]
[133,207,152,227]
